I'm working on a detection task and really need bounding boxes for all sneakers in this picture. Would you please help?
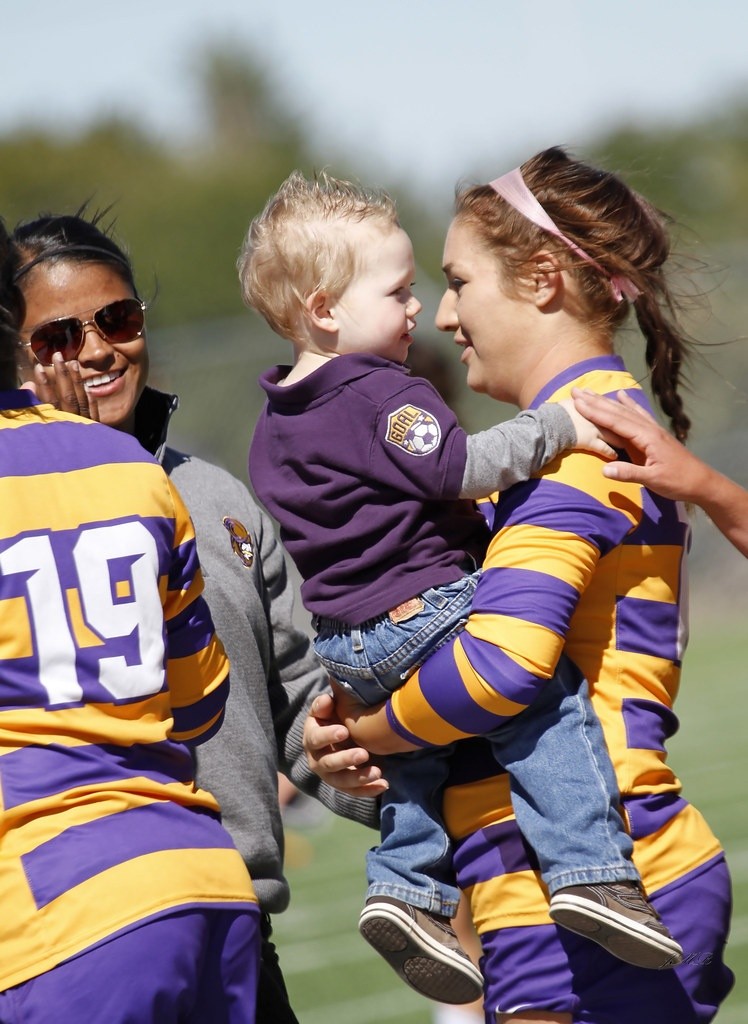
[357,896,485,1005]
[548,881,685,970]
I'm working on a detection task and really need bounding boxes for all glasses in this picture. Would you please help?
[17,298,147,368]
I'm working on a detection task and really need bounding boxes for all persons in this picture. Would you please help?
[568,387,747,558]
[7,215,376,1024]
[0,225,265,1024]
[239,164,684,1007]
[302,148,738,1024]
